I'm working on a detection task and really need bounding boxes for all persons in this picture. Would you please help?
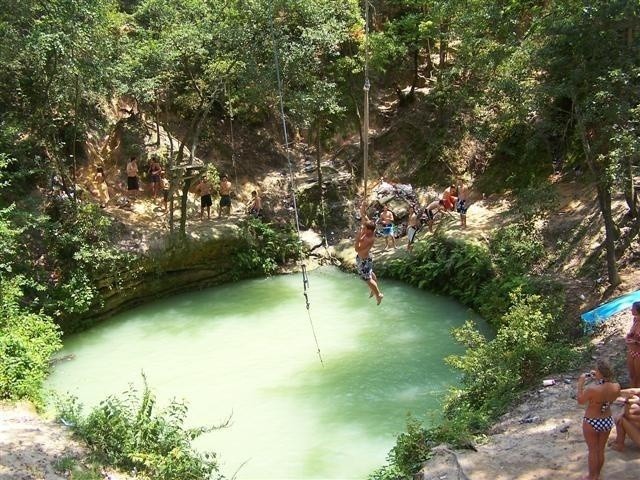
[355,207,384,306]
[193,176,261,221]
[379,183,467,254]
[68,156,170,214]
[576,302,640,480]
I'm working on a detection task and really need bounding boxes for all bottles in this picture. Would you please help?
[543,379,555,387]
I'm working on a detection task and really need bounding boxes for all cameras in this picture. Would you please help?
[585,373,593,378]
